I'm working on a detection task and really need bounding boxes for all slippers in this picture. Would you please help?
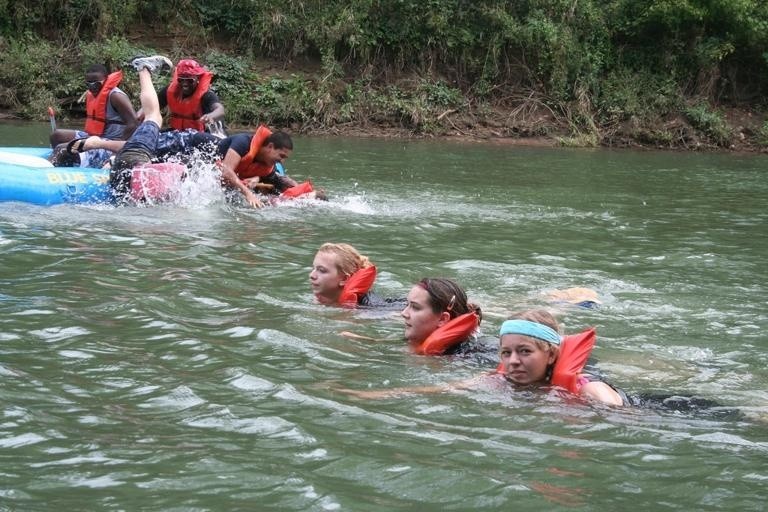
[67,138,91,155]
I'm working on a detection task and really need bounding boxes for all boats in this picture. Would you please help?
[0,146,283,205]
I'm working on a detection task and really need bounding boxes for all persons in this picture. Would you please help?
[394,279,497,359]
[49,53,326,209]
[339,309,756,425]
[308,240,389,313]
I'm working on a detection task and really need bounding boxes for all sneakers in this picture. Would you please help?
[131,54,173,74]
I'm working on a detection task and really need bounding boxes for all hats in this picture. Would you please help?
[175,60,204,76]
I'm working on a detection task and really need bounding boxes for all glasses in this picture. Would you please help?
[177,77,195,83]
[84,80,104,89]
